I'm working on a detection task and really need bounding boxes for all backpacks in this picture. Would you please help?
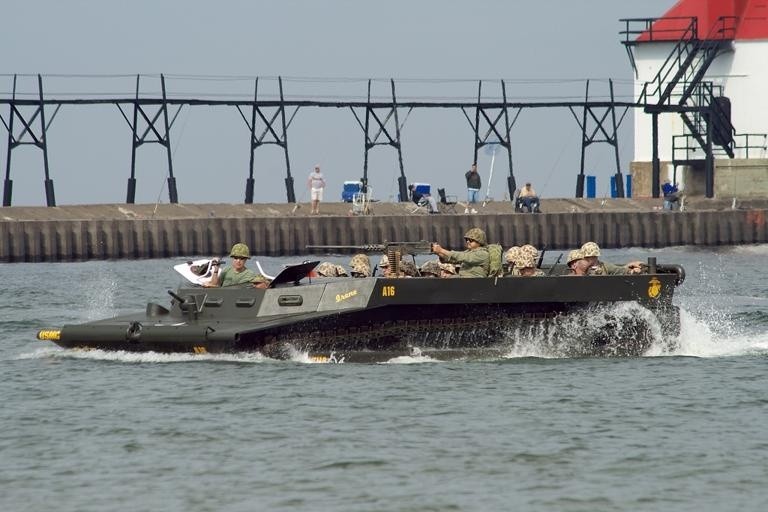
[487,243,504,277]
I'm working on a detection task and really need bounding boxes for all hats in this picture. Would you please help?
[228,244,251,260]
[504,242,602,270]
[464,227,488,246]
[317,253,455,277]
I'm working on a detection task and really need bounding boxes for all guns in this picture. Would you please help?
[371,264,378,278]
[537,245,548,269]
[306,240,435,274]
[548,254,563,276]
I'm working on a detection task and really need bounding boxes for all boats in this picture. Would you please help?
[36,256,685,362]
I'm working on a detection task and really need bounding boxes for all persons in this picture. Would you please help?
[567,242,647,275]
[313,227,547,277]
[407,184,441,214]
[518,183,543,212]
[307,165,327,215]
[464,164,482,216]
[210,243,270,288]
[662,178,689,212]
